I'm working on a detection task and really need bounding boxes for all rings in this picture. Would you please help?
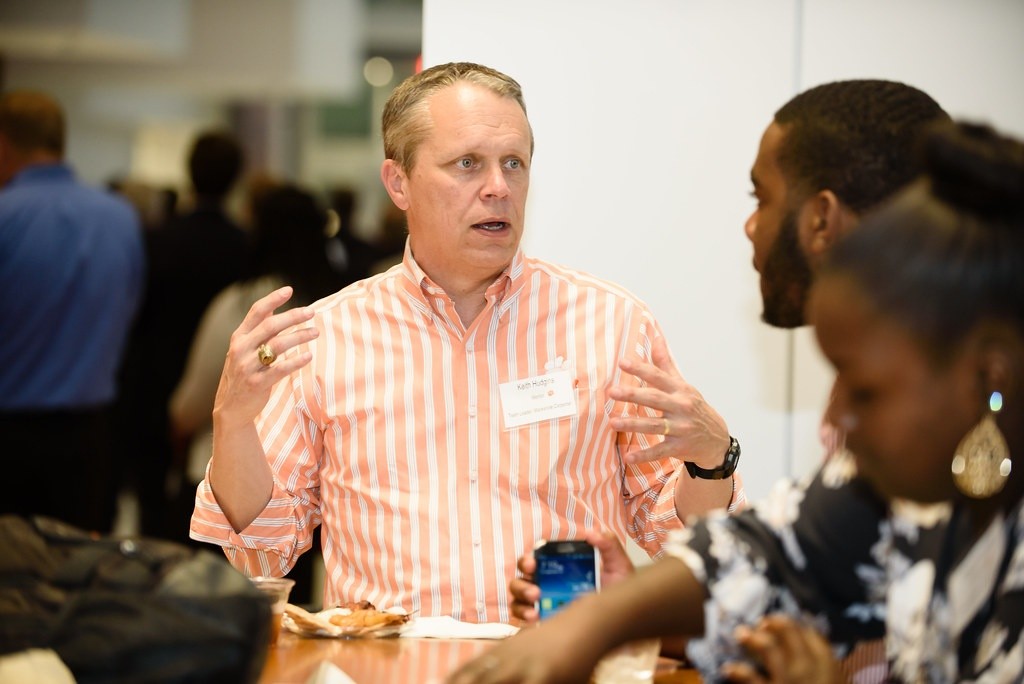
[258,343,276,366]
[661,419,671,436]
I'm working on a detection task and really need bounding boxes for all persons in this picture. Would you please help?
[444,81,954,684]
[189,62,745,627]
[722,120,1024,683]
[0,93,390,560]
[0,515,270,684]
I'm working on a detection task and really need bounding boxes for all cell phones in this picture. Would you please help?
[531,535,600,621]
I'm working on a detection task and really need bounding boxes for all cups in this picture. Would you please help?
[248,577,296,643]
[590,635,662,684]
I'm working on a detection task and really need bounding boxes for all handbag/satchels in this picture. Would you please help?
[0,514,287,684]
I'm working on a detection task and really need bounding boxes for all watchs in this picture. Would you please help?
[684,436,740,479]
